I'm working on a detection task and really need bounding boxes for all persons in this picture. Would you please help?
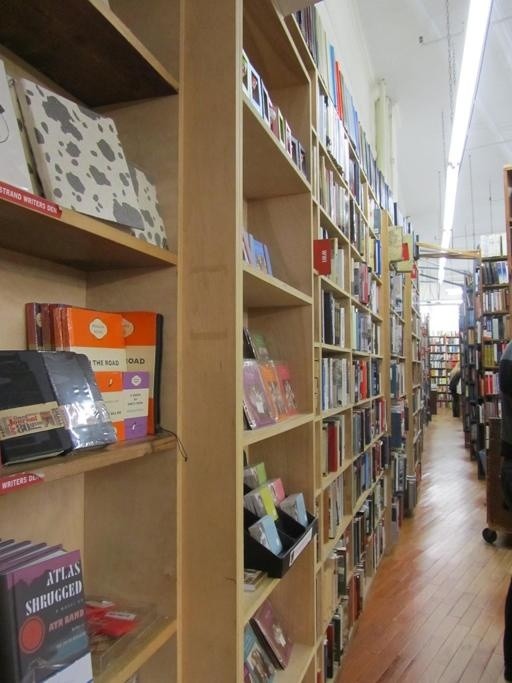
[448,368,461,418]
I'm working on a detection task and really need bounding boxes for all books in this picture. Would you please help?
[301,2,407,683]
[243,231,272,275]
[241,50,307,177]
[404,220,428,508]
[243,599,292,682]
[244,461,308,555]
[0,539,93,683]
[0,302,164,466]
[461,231,512,452]
[430,329,461,409]
[0,61,169,249]
[242,328,297,429]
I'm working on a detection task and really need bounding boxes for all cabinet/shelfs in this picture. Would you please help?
[1,0,428,683]
[459,165,512,549]
[428,335,460,409]
